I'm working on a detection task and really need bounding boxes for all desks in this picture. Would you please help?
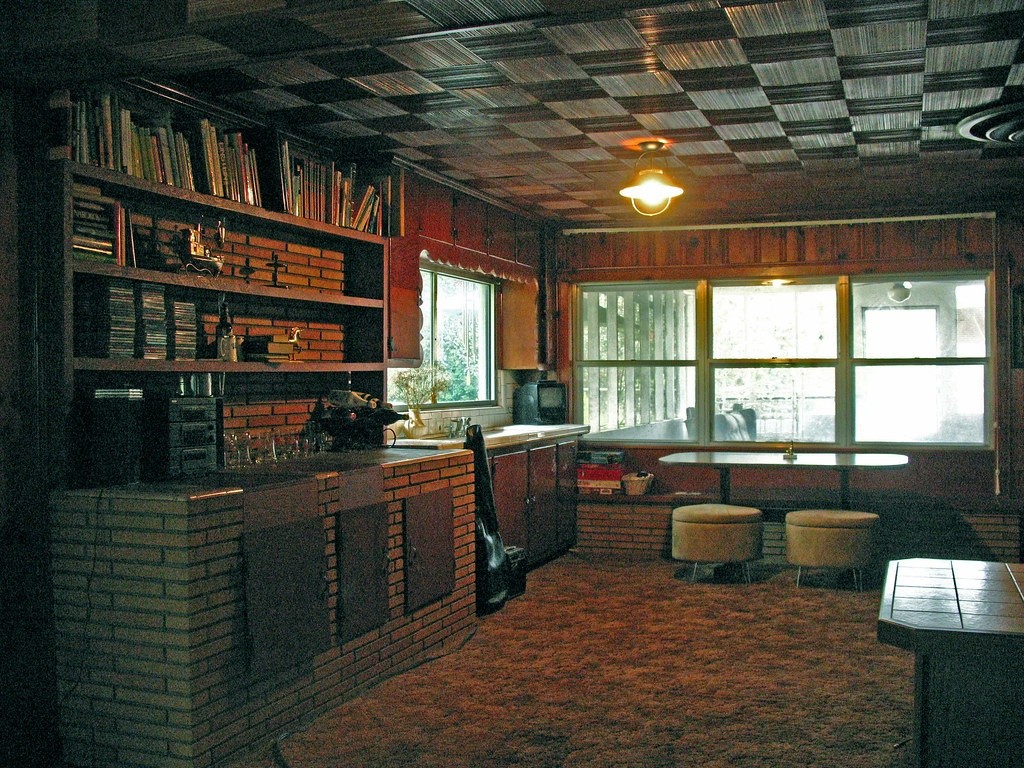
[877,557,1024,768]
[658,452,911,510]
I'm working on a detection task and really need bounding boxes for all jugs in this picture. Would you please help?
[188,371,226,399]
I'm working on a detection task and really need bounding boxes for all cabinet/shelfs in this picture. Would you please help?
[497,218,561,371]
[419,166,541,284]
[556,439,578,557]
[492,443,557,574]
[21,90,392,481]
[358,152,424,367]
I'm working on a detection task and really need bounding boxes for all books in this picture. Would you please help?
[71,184,136,264]
[72,94,391,235]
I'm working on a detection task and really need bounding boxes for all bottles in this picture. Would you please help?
[177,373,188,398]
[215,301,237,362]
[319,391,410,426]
[224,446,240,469]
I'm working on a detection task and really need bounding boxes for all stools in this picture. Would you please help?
[785,508,882,592]
[672,503,764,585]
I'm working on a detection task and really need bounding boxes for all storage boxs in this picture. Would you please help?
[577,450,626,496]
[622,473,654,496]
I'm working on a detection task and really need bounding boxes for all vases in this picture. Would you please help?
[402,408,427,439]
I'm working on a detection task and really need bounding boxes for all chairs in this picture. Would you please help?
[714,412,752,442]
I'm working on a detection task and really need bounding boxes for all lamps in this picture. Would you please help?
[619,140,683,217]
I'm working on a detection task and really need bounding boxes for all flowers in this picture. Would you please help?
[392,363,451,410]
